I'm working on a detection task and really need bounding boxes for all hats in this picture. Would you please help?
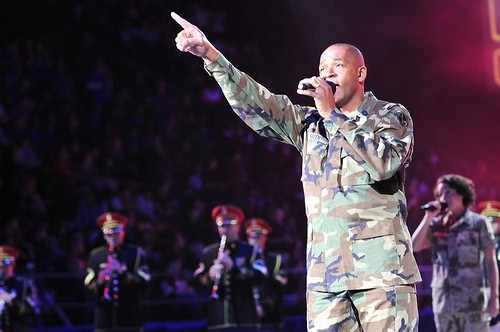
[242,218,272,236]
[96,212,128,235]
[211,204,245,227]
[0,245,20,267]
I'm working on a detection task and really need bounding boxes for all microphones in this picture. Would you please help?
[298,80,336,95]
[420,200,449,212]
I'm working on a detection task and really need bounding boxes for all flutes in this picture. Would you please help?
[212,235,228,303]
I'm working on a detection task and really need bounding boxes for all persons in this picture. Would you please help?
[0,0,306,300]
[84,211,151,332]
[168,9,424,331]
[0,246,37,332]
[479,202,500,255]
[192,205,267,332]
[412,173,500,332]
[240,217,289,332]
